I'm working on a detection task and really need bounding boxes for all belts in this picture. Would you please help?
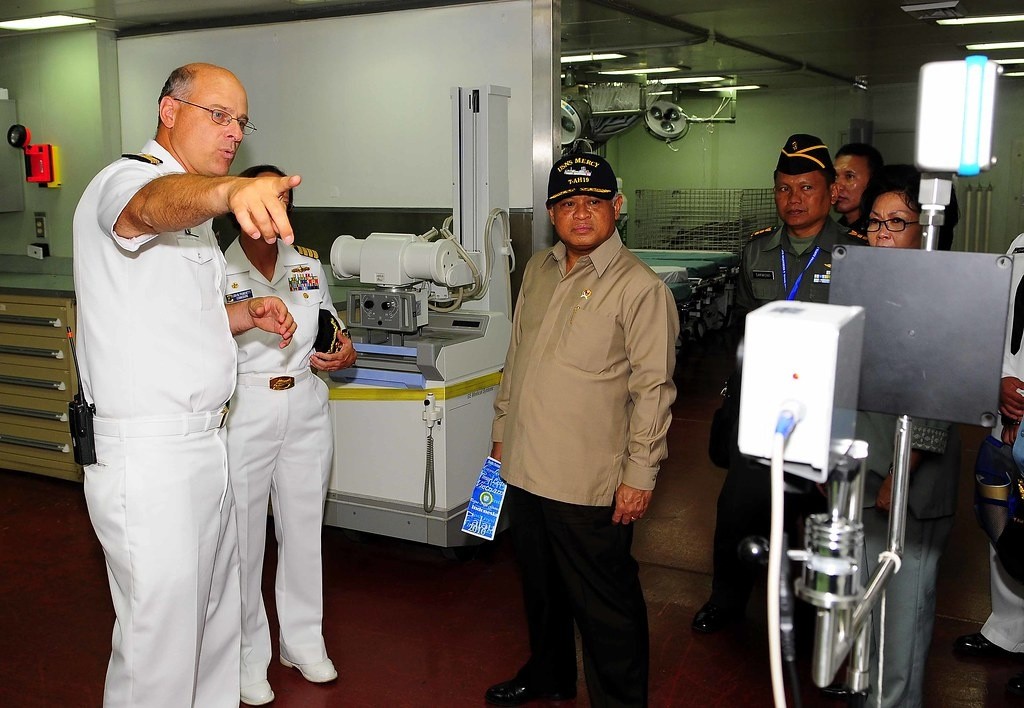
[92,411,228,438]
[236,368,312,390]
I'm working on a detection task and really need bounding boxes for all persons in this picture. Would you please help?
[831,142,883,235]
[72,63,301,708]
[225,165,358,705]
[859,163,960,708]
[951,232,1024,658]
[693,134,870,635]
[485,154,679,708]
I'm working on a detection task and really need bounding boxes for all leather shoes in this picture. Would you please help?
[690,593,749,632]
[1007,676,1024,695]
[485,654,578,705]
[953,631,1024,664]
[820,678,867,701]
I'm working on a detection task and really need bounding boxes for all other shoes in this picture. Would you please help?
[279,653,338,683]
[240,679,275,706]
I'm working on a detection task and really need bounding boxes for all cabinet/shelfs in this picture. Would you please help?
[0,270,85,484]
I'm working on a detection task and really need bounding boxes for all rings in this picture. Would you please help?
[631,517,636,520]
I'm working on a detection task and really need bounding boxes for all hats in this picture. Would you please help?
[777,134,834,175]
[547,154,618,207]
[311,306,341,375]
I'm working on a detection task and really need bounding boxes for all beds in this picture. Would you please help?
[629,250,741,359]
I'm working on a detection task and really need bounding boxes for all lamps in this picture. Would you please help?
[698,84,768,94]
[647,74,732,84]
[557,48,638,64]
[930,11,1024,78]
[8,124,55,183]
[592,63,692,75]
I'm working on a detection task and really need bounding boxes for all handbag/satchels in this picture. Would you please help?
[707,373,740,469]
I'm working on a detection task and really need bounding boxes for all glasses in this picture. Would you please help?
[861,216,919,232]
[172,98,258,135]
